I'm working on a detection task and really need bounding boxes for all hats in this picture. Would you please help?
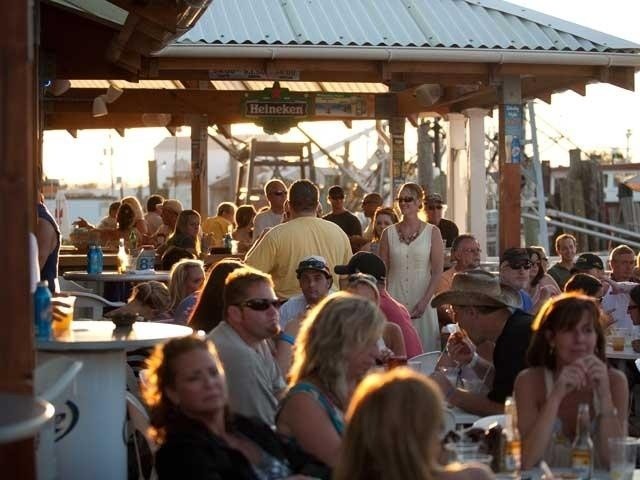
[328,185,345,200]
[335,250,387,281]
[501,246,534,268]
[155,198,184,216]
[567,253,604,277]
[295,258,333,280]
[425,192,446,206]
[429,269,522,310]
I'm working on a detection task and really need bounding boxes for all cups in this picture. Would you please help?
[49,296,78,331]
[606,434,640,479]
[610,328,628,352]
[443,440,496,471]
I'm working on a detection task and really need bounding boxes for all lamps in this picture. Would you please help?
[51,79,71,96]
[92,80,123,118]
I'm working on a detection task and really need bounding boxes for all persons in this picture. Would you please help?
[32,181,639,480]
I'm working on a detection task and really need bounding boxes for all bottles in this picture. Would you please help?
[499,395,523,476]
[32,279,53,340]
[87,238,127,276]
[571,404,595,480]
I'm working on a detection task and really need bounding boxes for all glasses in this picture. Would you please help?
[233,297,281,311]
[503,262,532,270]
[428,205,443,209]
[395,197,414,203]
[270,190,287,196]
